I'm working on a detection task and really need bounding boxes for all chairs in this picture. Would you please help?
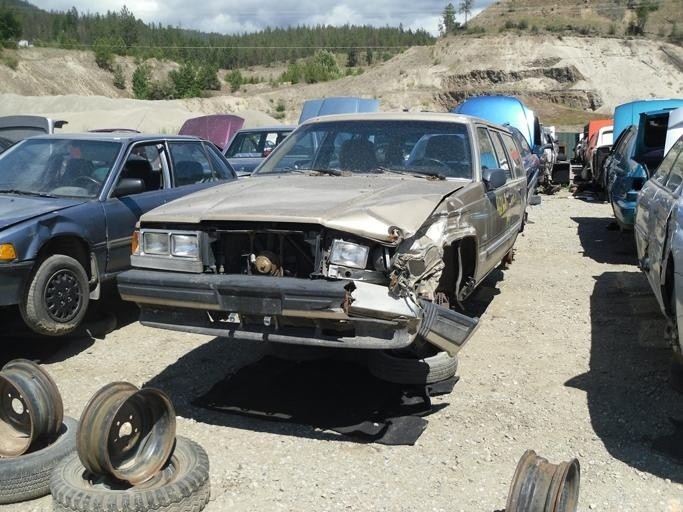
[125,160,151,183]
[427,135,471,177]
[341,139,379,171]
[59,158,92,185]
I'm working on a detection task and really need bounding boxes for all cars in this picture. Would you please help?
[633,134,682,362]
[117,111,529,352]
[1,132,241,337]
